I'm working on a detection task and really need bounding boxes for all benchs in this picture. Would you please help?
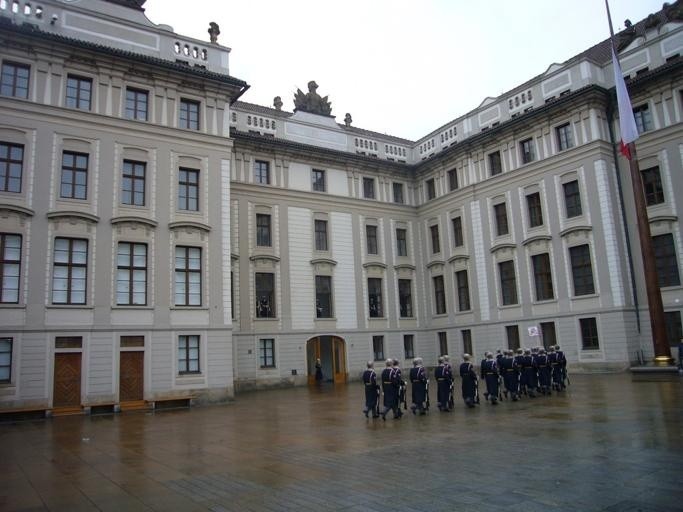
[79,401,120,414]
[144,395,197,410]
[0,405,52,418]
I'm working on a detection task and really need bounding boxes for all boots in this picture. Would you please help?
[484,391,519,405]
[363,409,403,421]
[465,398,479,408]
[411,405,426,415]
[517,382,566,398]
[436,402,455,412]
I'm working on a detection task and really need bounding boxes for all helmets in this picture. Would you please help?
[439,355,449,363]
[386,358,399,365]
[413,357,424,364]
[367,361,373,367]
[463,353,473,361]
[484,349,513,357]
[517,344,560,354]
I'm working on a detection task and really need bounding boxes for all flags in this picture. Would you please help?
[605,46,640,159]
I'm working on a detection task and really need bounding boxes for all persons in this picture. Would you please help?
[315,358,325,388]
[362,344,568,420]
[370,298,376,317]
[255,296,271,318]
[678,338,683,368]
[316,298,322,318]
